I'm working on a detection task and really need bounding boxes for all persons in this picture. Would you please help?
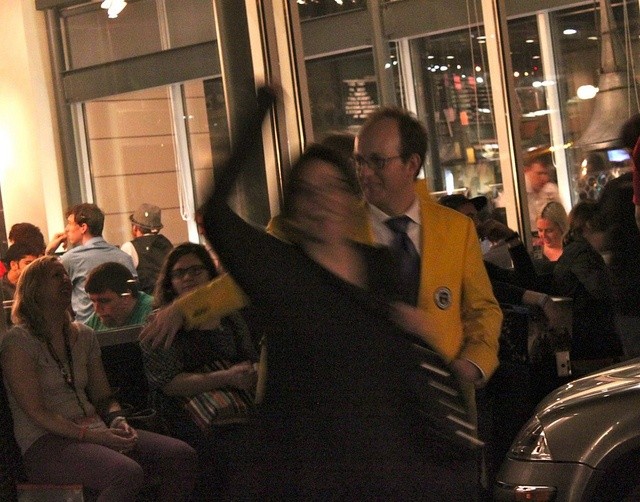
[0,256,195,502]
[585,115,640,360]
[81,260,153,335]
[530,201,569,275]
[139,109,506,439]
[579,154,616,204]
[553,203,628,370]
[121,204,174,295]
[143,242,260,458]
[1,241,38,301]
[9,222,44,249]
[45,204,138,326]
[201,82,417,500]
[433,194,580,369]
[523,158,559,251]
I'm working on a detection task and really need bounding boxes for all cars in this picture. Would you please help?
[494,354,639,501]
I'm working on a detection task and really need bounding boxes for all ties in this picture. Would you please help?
[385,216,421,308]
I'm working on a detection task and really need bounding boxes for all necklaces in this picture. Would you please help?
[40,324,87,419]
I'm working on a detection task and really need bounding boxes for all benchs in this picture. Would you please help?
[0,324,151,502]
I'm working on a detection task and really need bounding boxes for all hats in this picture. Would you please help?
[129,203,163,230]
[438,194,487,210]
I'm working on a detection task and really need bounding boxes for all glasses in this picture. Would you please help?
[167,265,209,278]
[351,153,409,170]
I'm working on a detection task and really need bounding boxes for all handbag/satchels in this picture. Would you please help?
[98,388,170,437]
[176,358,259,437]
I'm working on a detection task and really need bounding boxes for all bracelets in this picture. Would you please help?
[504,232,518,243]
[537,293,551,309]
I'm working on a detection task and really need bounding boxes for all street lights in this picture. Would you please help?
[576,81,640,100]
